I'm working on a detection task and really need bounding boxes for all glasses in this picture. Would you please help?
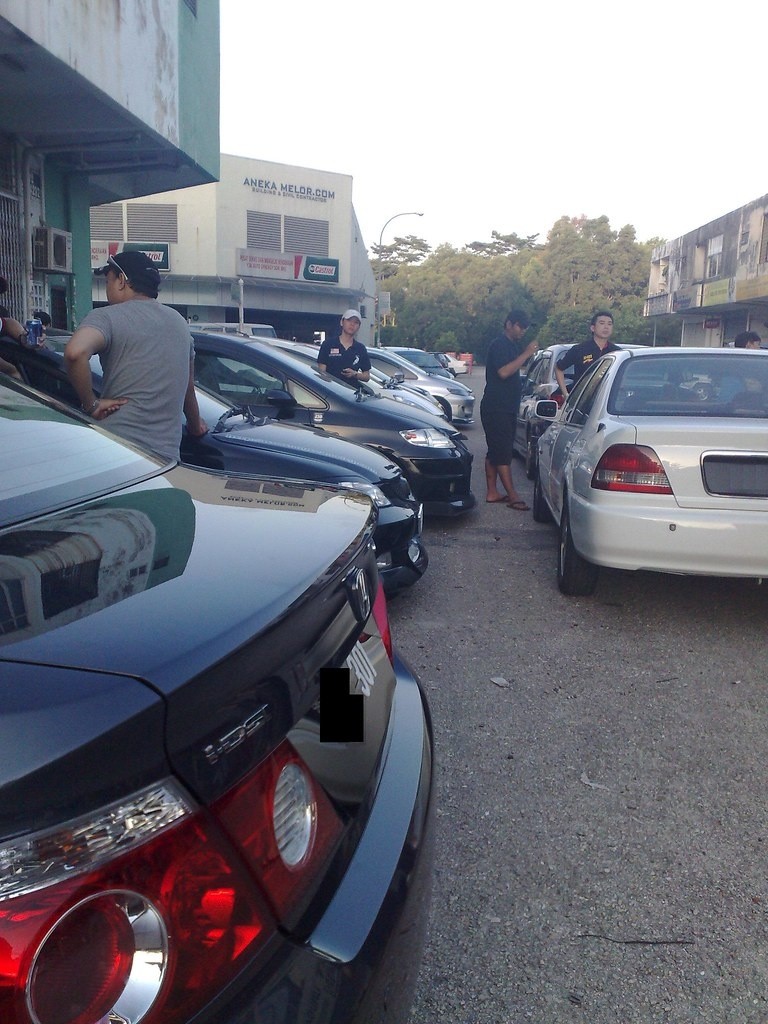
[107,255,128,280]
[512,322,527,330]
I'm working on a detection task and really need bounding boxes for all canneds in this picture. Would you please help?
[26,319,42,346]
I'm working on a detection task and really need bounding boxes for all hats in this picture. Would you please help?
[94,251,161,289]
[342,310,361,323]
[507,310,537,328]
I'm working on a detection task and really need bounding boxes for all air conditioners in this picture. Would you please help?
[33,227,73,275]
[360,305,368,320]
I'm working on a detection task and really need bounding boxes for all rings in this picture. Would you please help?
[534,346,537,348]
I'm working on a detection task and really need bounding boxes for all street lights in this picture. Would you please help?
[377,211,426,351]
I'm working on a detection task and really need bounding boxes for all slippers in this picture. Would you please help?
[486,495,508,503]
[506,499,531,511]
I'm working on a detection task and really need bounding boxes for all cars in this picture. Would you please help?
[0,318,431,601]
[189,317,477,429]
[193,327,479,520]
[512,343,720,485]
[1,368,438,1024]
[532,345,767,598]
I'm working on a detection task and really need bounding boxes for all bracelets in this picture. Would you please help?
[80,399,98,415]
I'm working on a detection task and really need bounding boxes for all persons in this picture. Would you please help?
[0,277,50,380]
[554,313,621,397]
[63,250,207,458]
[734,332,761,349]
[317,310,371,384]
[480,310,539,509]
